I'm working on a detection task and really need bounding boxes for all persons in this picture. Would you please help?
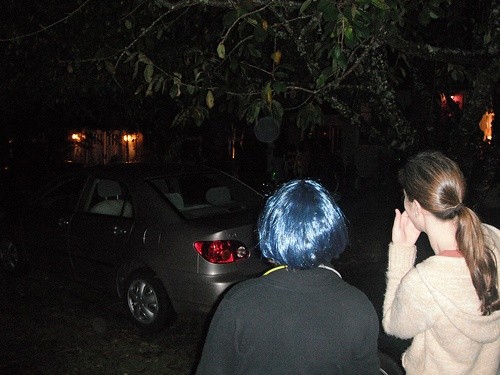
[196,178,383,374]
[379,150,500,374]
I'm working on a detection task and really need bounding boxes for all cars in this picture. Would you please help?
[0,162,273,335]
